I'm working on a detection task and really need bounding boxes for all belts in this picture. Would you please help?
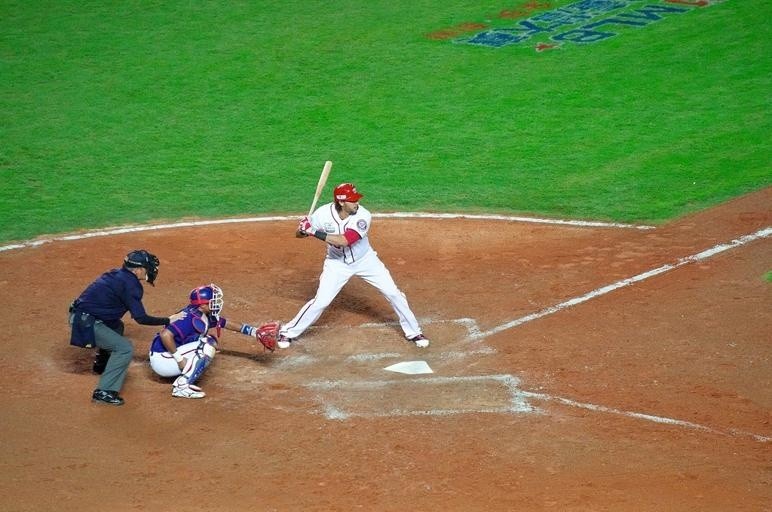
[70,303,100,320]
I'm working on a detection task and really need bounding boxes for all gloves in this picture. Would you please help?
[298,218,316,234]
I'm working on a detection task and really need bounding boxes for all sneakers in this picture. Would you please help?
[172,384,205,399]
[413,334,429,347]
[278,335,291,348]
[92,389,124,405]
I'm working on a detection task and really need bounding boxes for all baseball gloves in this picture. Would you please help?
[257,322,279,351]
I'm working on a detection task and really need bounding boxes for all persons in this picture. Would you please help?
[66,249,187,406]
[276,181,430,350]
[148,283,281,401]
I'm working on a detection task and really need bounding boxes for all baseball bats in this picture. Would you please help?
[307,161,332,219]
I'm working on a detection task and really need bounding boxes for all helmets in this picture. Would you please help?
[124,250,160,287]
[190,284,223,315]
[334,183,364,202]
